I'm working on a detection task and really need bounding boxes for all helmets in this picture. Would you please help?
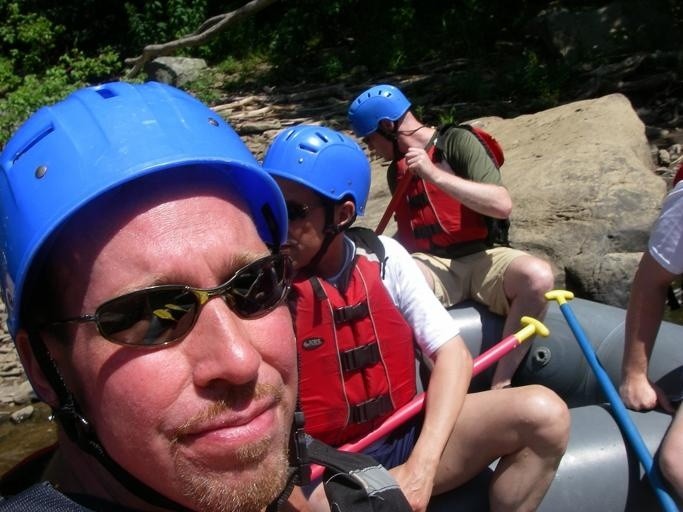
[0,80,289,341]
[261,125,371,216]
[350,85,412,137]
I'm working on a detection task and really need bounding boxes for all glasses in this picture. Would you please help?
[362,133,374,145]
[30,252,296,350]
[286,199,324,221]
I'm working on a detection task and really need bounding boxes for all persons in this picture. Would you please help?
[618,178,682,505]
[0,79,414,510]
[258,119,570,511]
[345,84,556,390]
